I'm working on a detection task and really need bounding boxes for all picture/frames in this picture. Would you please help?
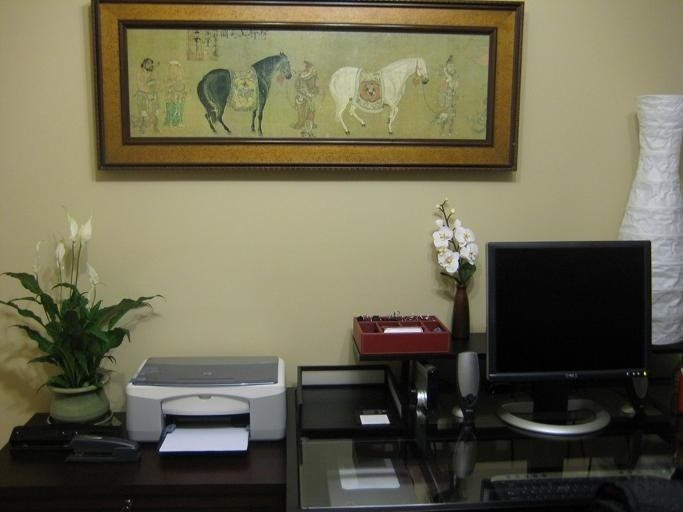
[91,0,524,173]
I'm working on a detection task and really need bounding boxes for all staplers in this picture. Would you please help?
[68,435,141,463]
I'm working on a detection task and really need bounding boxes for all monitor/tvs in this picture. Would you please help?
[486,240,653,438]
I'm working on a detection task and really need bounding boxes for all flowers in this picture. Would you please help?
[0,210,166,391]
[431,199,480,284]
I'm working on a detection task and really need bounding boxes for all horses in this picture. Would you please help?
[327,54,430,136]
[196,51,292,137]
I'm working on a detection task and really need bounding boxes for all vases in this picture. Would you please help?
[451,280,471,342]
[47,373,118,429]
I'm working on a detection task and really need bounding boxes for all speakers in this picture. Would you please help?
[451,350,482,419]
[620,375,650,415]
[447,423,479,482]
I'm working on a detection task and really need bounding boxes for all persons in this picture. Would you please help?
[288,60,321,139]
[434,56,460,138]
[135,57,164,134]
[160,58,191,132]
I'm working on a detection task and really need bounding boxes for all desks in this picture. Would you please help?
[0,411,287,512]
[292,381,683,512]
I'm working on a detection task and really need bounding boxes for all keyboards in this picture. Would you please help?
[479,473,635,512]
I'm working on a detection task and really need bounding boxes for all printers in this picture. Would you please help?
[124,354,288,457]
